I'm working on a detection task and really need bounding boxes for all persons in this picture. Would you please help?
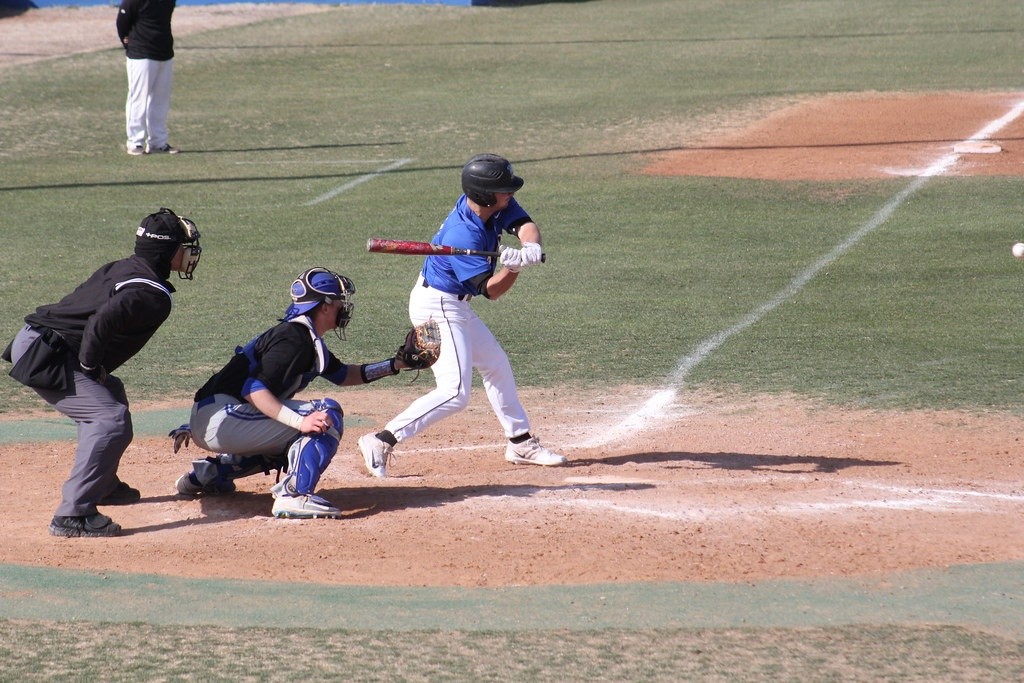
[358,152,567,477]
[1,207,202,538]
[116,0,179,155]
[176,266,441,519]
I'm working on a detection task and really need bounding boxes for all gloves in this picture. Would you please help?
[519,241,542,268]
[499,245,523,274]
[169,424,193,455]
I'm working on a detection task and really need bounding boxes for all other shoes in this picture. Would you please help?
[128,148,147,156]
[146,142,178,153]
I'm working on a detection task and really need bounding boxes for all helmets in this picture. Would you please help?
[284,270,340,316]
[461,153,524,207]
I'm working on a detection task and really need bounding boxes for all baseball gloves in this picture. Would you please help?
[392,318,442,384]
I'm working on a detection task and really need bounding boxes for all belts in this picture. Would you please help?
[422,280,466,301]
[198,396,216,409]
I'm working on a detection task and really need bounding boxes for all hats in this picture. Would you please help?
[135,211,195,243]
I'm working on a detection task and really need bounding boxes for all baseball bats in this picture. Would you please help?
[365,237,548,263]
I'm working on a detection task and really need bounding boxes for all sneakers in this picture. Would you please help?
[505,440,566,468]
[358,433,397,478]
[271,492,342,519]
[176,473,236,496]
[98,484,141,506]
[50,512,121,536]
